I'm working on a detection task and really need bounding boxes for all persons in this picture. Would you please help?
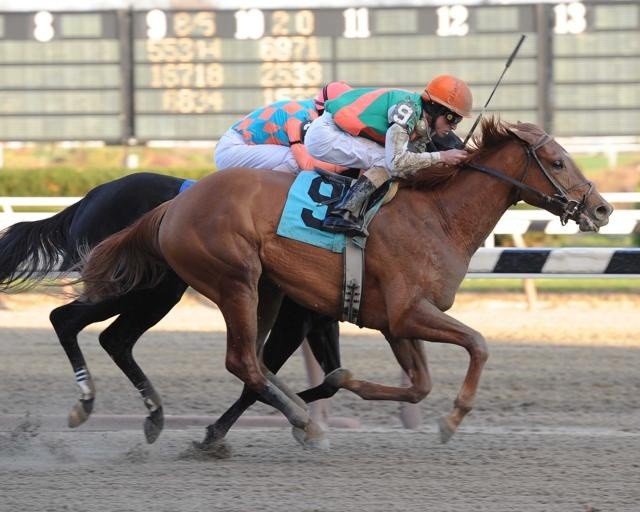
[302,73,475,241]
[212,79,353,175]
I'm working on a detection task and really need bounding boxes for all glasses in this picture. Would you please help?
[431,102,463,125]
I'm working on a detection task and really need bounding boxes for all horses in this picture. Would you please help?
[41,108,617,452]
[0,130,468,449]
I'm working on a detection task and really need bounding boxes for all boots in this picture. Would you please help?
[322,172,377,238]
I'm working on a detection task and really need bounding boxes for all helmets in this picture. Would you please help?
[313,81,353,110]
[420,74,474,118]
